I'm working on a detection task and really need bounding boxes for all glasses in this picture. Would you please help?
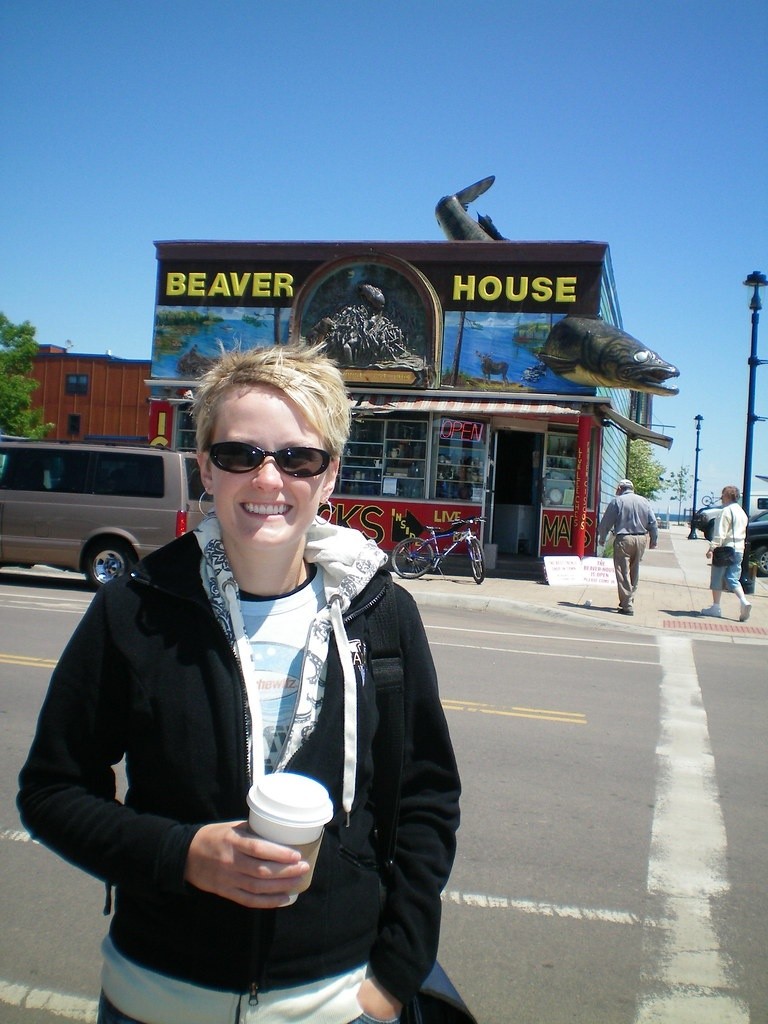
[207,442,332,479]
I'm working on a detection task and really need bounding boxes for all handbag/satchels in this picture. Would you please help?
[400,960,479,1024]
[713,546,736,567]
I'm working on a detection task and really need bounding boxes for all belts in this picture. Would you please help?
[617,532,644,535]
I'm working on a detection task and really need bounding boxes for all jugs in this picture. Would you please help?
[373,460,380,467]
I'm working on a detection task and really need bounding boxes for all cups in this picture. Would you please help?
[245,772,335,897]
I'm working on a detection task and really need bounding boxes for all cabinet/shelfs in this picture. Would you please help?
[433,421,488,500]
[544,432,578,508]
[338,415,427,497]
[176,413,199,451]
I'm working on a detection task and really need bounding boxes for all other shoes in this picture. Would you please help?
[619,609,633,615]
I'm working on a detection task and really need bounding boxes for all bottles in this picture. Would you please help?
[409,462,416,477]
[391,448,398,458]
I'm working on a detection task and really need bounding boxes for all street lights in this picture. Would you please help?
[687,414,705,538]
[739,269,768,595]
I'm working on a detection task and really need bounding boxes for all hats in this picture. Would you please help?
[615,479,634,495]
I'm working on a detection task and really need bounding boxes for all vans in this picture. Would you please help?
[0,434,212,591]
[698,490,768,542]
[693,496,728,532]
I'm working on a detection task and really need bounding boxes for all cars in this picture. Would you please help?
[744,509,768,577]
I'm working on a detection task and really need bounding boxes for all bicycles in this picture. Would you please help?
[391,514,487,585]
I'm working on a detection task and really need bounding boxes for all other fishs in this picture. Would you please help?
[434,173,683,399]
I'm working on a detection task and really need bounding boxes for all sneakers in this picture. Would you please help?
[701,608,721,617]
[740,602,751,621]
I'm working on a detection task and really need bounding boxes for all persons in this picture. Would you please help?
[598,479,660,617]
[700,486,752,622]
[16,338,462,1024]
